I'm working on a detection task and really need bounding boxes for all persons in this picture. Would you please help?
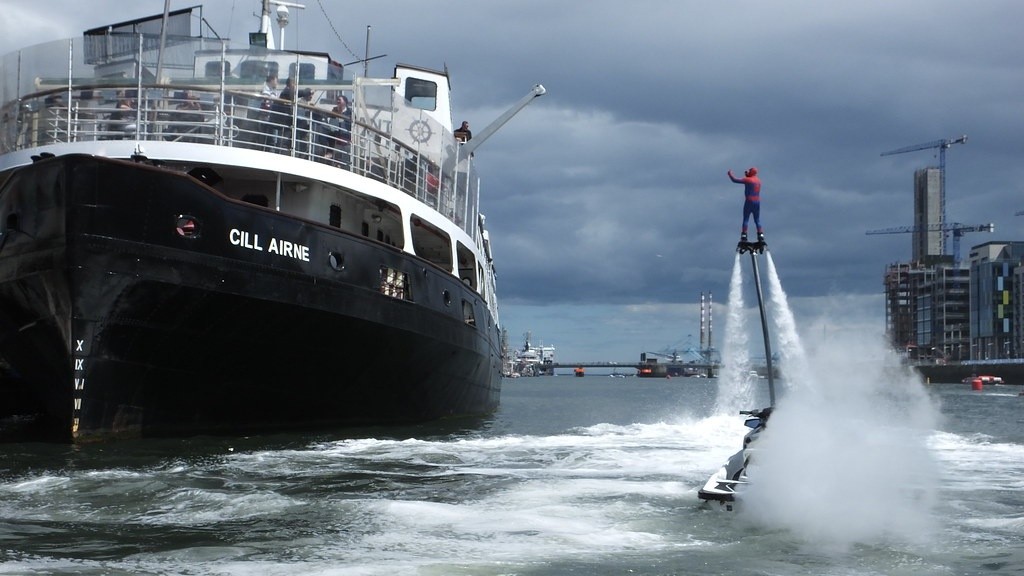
[324,94,357,159]
[453,120,473,147]
[258,74,316,159]
[109,87,204,142]
[727,167,767,240]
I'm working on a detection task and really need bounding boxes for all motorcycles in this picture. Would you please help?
[697,407,776,515]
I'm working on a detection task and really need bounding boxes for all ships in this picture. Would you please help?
[0,0,547,442]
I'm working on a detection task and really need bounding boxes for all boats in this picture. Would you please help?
[960,374,1004,384]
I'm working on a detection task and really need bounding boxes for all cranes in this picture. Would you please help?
[865,135,997,292]
